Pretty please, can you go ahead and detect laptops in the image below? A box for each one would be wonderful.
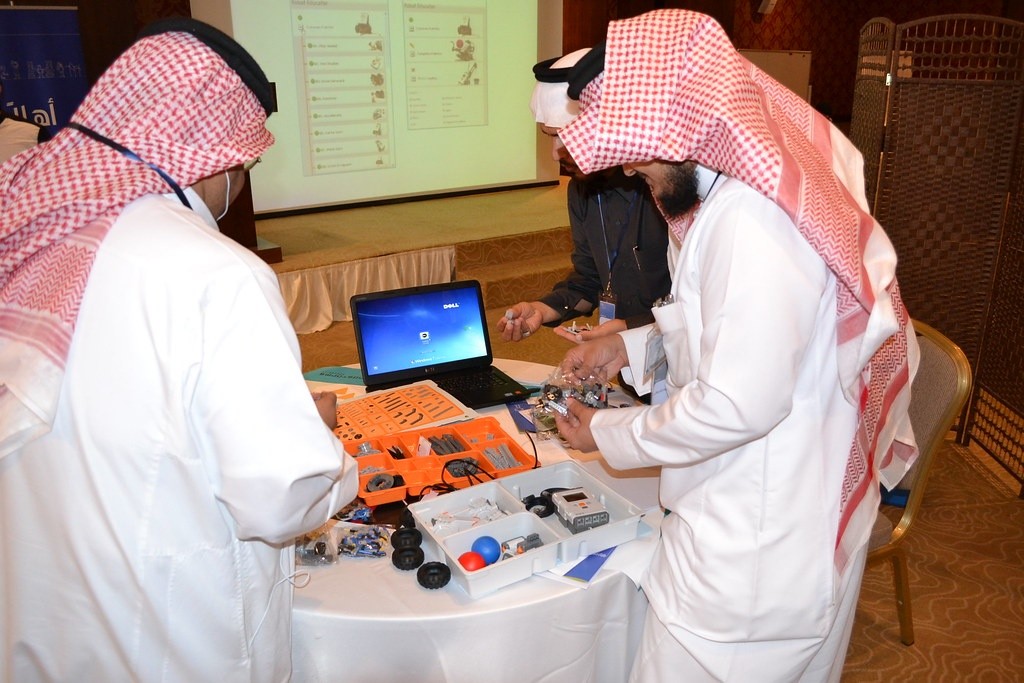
[349,280,531,410]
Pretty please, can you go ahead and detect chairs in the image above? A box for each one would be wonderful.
[862,319,972,650]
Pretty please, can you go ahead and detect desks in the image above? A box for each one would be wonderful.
[286,357,663,683]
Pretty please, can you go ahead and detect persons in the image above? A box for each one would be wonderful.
[0,15,359,683]
[498,41,673,344]
[556,9,920,683]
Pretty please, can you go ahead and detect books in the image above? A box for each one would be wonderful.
[534,546,617,590]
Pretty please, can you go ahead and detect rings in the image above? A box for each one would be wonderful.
[521,329,532,337]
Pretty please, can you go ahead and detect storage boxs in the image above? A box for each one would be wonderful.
[342,415,541,507]
[406,459,645,597]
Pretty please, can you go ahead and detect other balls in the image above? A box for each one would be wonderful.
[458,551,486,571]
[472,536,501,565]
